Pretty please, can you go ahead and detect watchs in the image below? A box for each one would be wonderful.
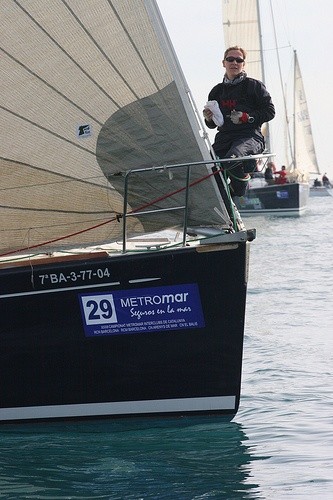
[247,113,255,123]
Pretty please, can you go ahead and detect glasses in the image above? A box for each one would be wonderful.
[225,56,245,63]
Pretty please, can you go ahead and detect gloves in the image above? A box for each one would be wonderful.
[230,110,249,125]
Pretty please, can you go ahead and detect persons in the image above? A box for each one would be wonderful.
[265,163,275,185]
[314,178,321,185]
[272,166,287,184]
[322,173,328,185]
[202,45,276,198]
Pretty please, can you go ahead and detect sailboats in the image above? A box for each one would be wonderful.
[0,0,333,428]
